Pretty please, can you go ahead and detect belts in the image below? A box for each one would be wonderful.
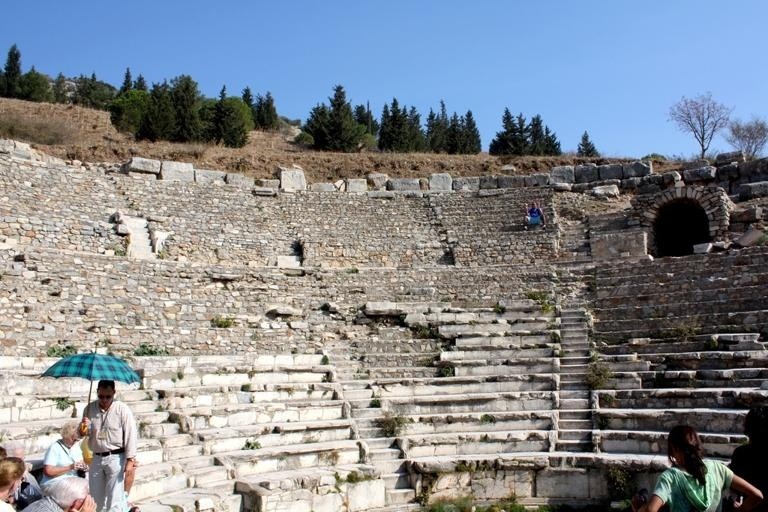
[96,447,125,456]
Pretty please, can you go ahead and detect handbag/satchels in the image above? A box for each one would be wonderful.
[17,482,42,510]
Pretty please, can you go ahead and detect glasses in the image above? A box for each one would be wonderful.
[98,394,113,399]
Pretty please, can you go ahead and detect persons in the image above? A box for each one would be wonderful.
[524,200,547,231]
[728,404,768,511]
[628,495,648,512]
[647,423,764,512]
[0,379,139,512]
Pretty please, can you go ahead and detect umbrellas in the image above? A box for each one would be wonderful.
[39,345,143,435]
